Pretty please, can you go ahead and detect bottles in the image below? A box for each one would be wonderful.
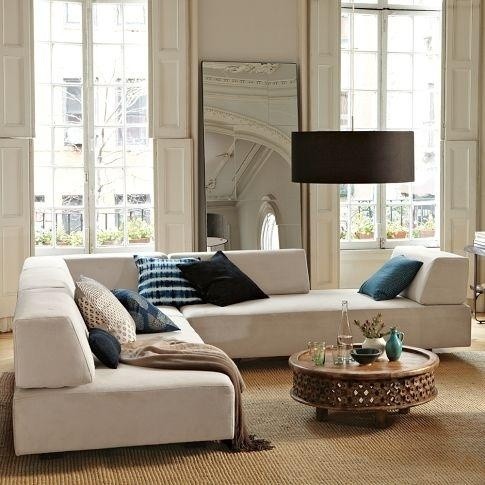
[338,301,352,362]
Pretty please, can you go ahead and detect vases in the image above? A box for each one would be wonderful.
[361,338,387,358]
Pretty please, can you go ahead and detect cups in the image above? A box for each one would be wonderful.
[308,339,323,361]
[344,349,356,364]
[314,342,326,366]
[331,346,346,365]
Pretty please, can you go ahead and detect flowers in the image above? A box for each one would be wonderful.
[352,313,398,338]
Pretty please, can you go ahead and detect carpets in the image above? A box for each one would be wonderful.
[0,351,485,485]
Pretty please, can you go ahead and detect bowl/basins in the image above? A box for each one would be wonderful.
[349,348,382,364]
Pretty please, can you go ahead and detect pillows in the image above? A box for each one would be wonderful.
[174,250,272,308]
[131,255,207,307]
[106,287,182,336]
[356,253,425,303]
[74,273,138,345]
[86,326,122,370]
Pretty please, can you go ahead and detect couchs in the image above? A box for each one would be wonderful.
[13,251,237,461]
[169,246,473,361]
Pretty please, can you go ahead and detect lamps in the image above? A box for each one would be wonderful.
[207,211,230,247]
[290,1,416,186]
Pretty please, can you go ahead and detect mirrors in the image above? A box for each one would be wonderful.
[195,57,311,291]
